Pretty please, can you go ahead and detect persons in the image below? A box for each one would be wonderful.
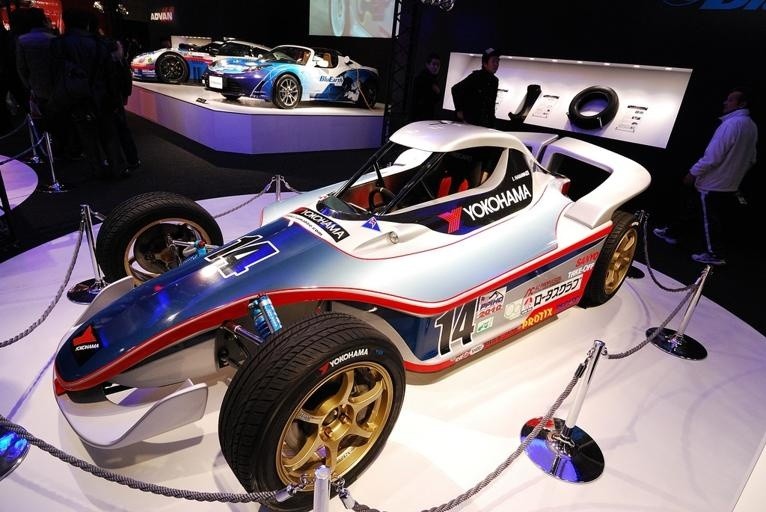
[406,54,443,125]
[652,87,759,267]
[45,7,142,179]
[451,46,503,123]
[13,6,61,162]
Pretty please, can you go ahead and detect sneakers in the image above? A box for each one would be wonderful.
[692,252,728,265]
[653,227,678,245]
[119,159,144,177]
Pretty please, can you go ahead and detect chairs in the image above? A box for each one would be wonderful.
[301,50,334,68]
[419,147,482,203]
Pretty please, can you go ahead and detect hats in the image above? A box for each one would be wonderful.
[483,48,502,64]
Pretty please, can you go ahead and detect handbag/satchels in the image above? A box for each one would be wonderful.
[104,57,132,98]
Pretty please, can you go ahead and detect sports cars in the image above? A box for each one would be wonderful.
[129,37,379,110]
[53,120,652,512]
